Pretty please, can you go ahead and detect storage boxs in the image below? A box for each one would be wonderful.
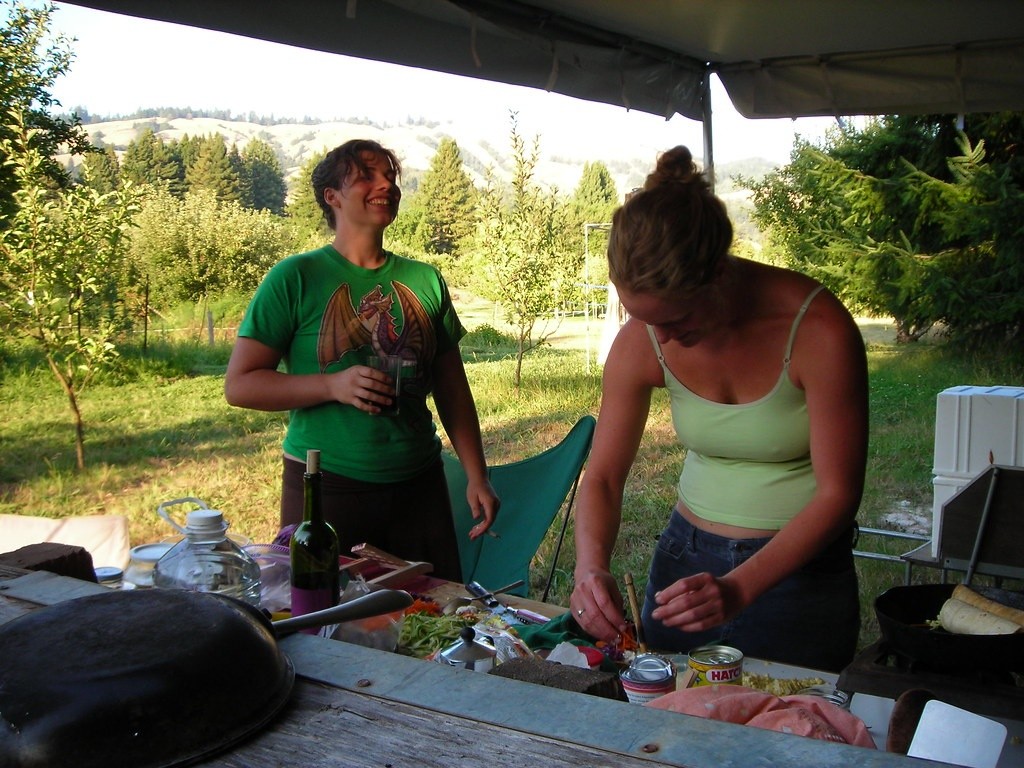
[932,385,1024,562]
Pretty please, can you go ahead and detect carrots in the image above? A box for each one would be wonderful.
[356,598,439,629]
[595,623,636,653]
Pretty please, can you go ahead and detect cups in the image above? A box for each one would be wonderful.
[370,354,404,416]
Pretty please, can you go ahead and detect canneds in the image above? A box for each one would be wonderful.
[618,652,677,705]
[686,645,744,688]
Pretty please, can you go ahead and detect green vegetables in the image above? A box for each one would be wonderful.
[393,608,475,659]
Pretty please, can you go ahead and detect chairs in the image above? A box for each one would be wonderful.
[437,414,595,602]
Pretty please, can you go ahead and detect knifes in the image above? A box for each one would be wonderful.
[464,579,522,623]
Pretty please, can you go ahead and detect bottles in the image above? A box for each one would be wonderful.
[152,497,262,611]
[290,448,342,635]
[95,566,123,589]
[122,543,175,589]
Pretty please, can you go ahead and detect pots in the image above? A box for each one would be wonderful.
[0,588,416,767]
[874,584,1024,680]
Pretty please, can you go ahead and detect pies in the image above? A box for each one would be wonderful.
[938,583,1024,634]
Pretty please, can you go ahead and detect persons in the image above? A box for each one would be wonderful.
[569,146,868,672]
[224,139,501,584]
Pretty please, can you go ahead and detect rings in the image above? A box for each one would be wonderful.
[577,609,585,617]
